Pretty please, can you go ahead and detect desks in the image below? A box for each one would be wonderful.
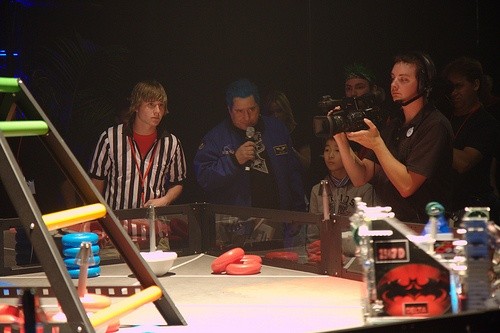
[0,201,500,333]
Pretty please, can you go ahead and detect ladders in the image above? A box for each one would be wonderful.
[0,75,189,331]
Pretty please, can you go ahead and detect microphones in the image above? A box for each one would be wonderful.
[395,93,425,107]
[245,127,255,171]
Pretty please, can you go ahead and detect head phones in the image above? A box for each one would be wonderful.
[415,52,434,93]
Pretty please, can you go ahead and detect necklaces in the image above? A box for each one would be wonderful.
[454,105,479,139]
[127,133,158,207]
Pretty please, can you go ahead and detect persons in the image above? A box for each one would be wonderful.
[261,90,311,173]
[193,79,308,250]
[5,65,76,209]
[327,50,455,224]
[90,78,186,240]
[443,57,499,226]
[309,136,379,255]
[344,64,391,160]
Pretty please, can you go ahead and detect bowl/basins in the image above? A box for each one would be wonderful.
[139,252,176,276]
